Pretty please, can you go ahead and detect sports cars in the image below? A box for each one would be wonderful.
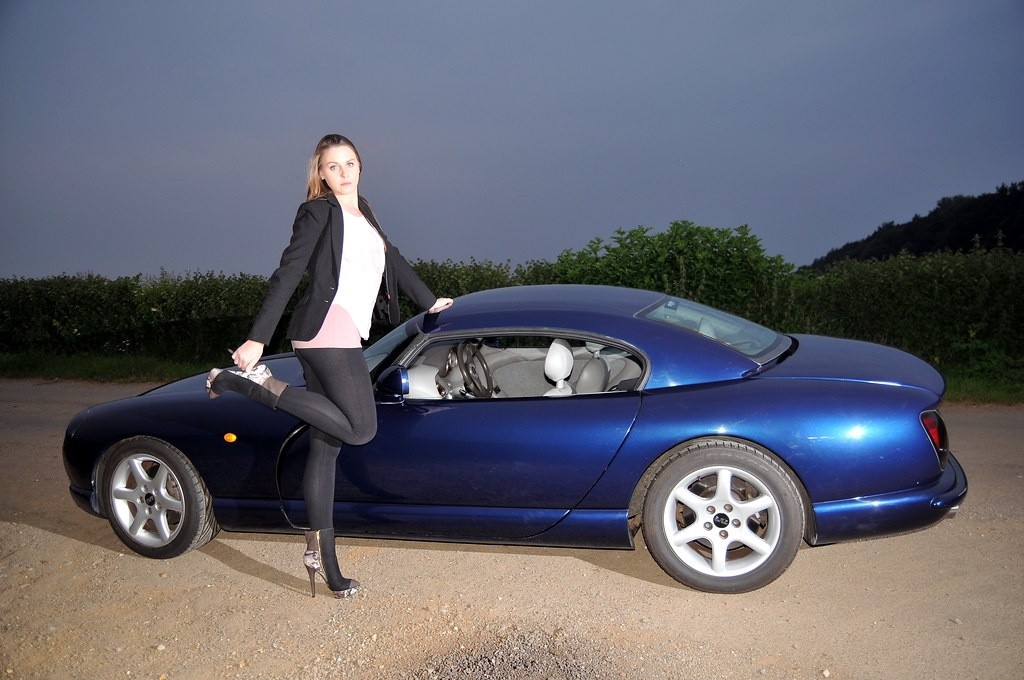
[58,284,970,594]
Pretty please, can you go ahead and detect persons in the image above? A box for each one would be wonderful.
[204,135,454,595]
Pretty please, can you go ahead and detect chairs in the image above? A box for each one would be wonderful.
[575,342,609,393]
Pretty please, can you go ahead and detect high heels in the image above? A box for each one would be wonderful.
[205,348,289,411]
[305,528,360,599]
